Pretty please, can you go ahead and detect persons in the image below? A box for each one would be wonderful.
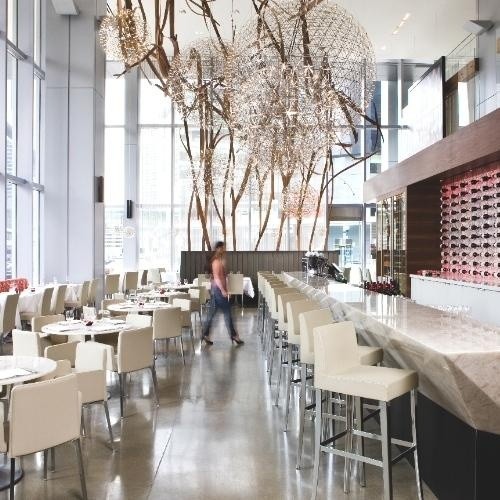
[200,240,244,345]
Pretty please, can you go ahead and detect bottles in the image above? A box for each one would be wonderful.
[438,172,500,279]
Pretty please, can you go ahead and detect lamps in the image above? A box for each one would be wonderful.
[97,174,105,203]
[127,199,132,218]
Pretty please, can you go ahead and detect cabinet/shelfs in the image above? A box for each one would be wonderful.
[376,185,442,298]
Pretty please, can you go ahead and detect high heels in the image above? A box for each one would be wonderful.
[201,335,213,345]
[231,333,245,345]
